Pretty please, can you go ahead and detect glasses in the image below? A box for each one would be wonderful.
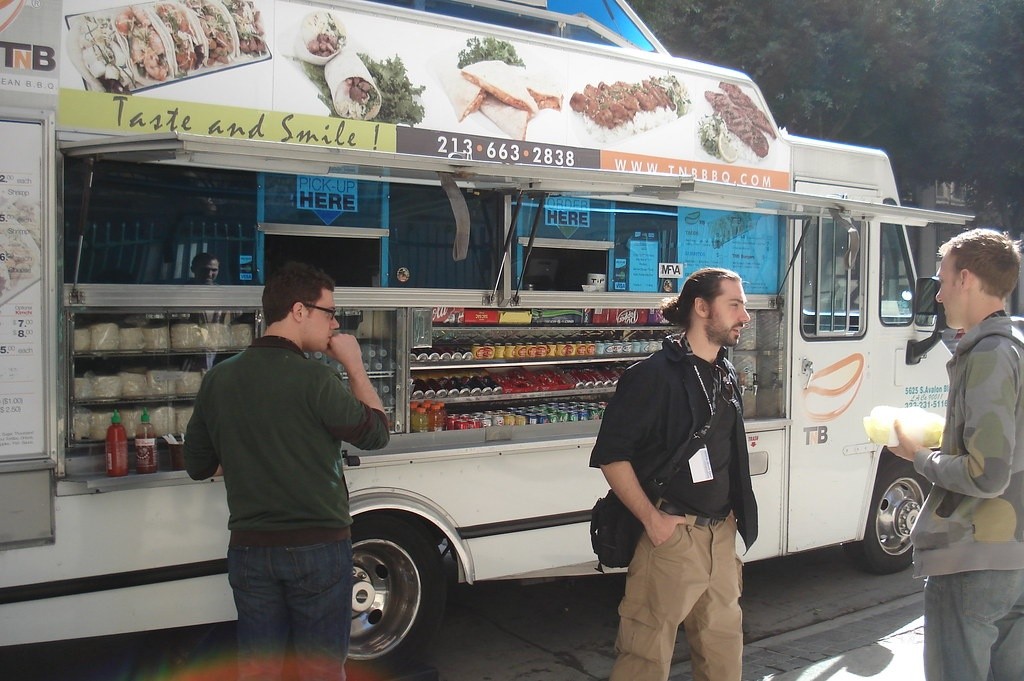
[302,303,337,320]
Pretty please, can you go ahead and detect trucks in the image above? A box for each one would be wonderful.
[1,0,1019,667]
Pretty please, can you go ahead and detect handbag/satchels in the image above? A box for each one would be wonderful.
[590,487,648,573]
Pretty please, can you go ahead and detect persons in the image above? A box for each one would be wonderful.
[590,268,760,681]
[175,255,232,372]
[182,264,391,681]
[885,227,1024,680]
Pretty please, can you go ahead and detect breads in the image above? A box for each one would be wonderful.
[443,55,562,142]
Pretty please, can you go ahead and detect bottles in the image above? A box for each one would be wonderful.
[105,408,128,478]
[303,342,397,410]
[134,407,158,474]
[409,400,445,434]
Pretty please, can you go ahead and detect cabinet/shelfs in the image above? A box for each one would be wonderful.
[62,309,404,458]
[404,304,684,439]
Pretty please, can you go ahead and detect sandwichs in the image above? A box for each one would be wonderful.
[65,0,266,93]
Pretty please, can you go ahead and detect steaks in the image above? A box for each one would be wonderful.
[706,79,776,158]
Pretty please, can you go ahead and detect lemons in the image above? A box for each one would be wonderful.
[716,132,738,163]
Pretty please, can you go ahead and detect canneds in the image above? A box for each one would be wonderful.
[411,333,677,437]
